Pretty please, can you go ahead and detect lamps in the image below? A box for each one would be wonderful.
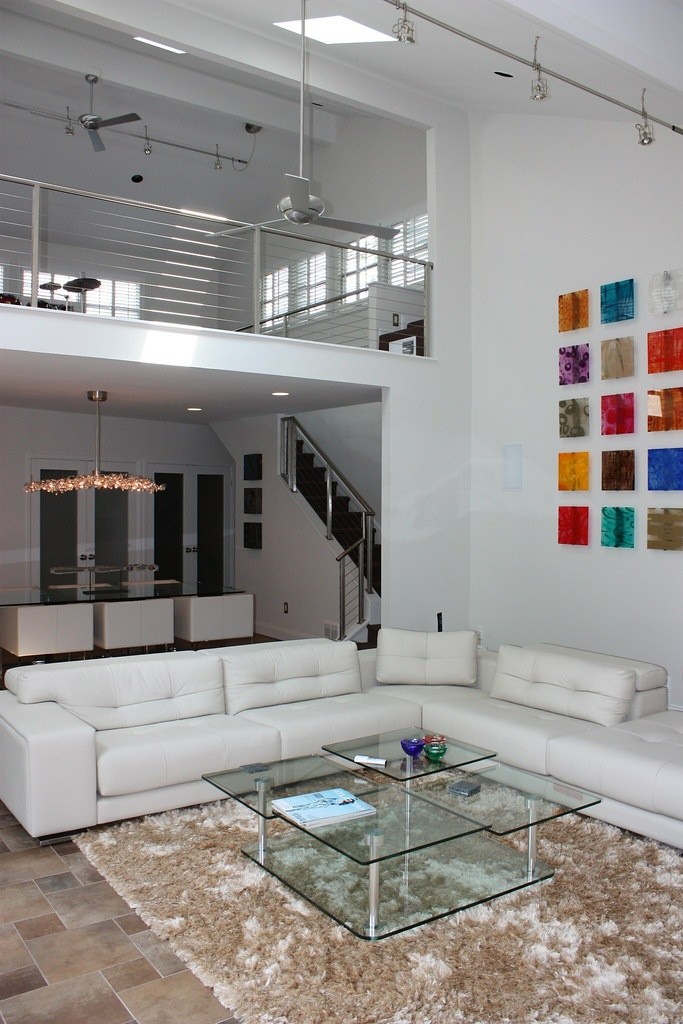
[64,107,74,136]
[529,35,549,101]
[392,0,415,44]
[144,126,152,154]
[215,144,222,170]
[635,89,656,145]
[23,391,167,494]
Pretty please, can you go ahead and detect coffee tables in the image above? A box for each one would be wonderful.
[201,725,601,942]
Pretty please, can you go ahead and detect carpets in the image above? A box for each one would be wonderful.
[71,756,683,1024]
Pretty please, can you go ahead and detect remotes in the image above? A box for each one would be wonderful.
[354,756,387,765]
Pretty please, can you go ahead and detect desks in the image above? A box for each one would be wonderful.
[0,582,245,606]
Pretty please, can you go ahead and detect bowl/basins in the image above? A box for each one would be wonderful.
[400,735,448,761]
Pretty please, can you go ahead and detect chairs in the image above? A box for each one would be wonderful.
[93,599,174,659]
[172,593,254,650]
[0,603,94,665]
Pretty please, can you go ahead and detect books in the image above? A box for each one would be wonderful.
[270,788,377,829]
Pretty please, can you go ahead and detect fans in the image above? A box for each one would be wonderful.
[31,75,141,152]
[205,1,399,240]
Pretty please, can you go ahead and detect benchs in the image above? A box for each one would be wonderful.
[0,627,683,852]
